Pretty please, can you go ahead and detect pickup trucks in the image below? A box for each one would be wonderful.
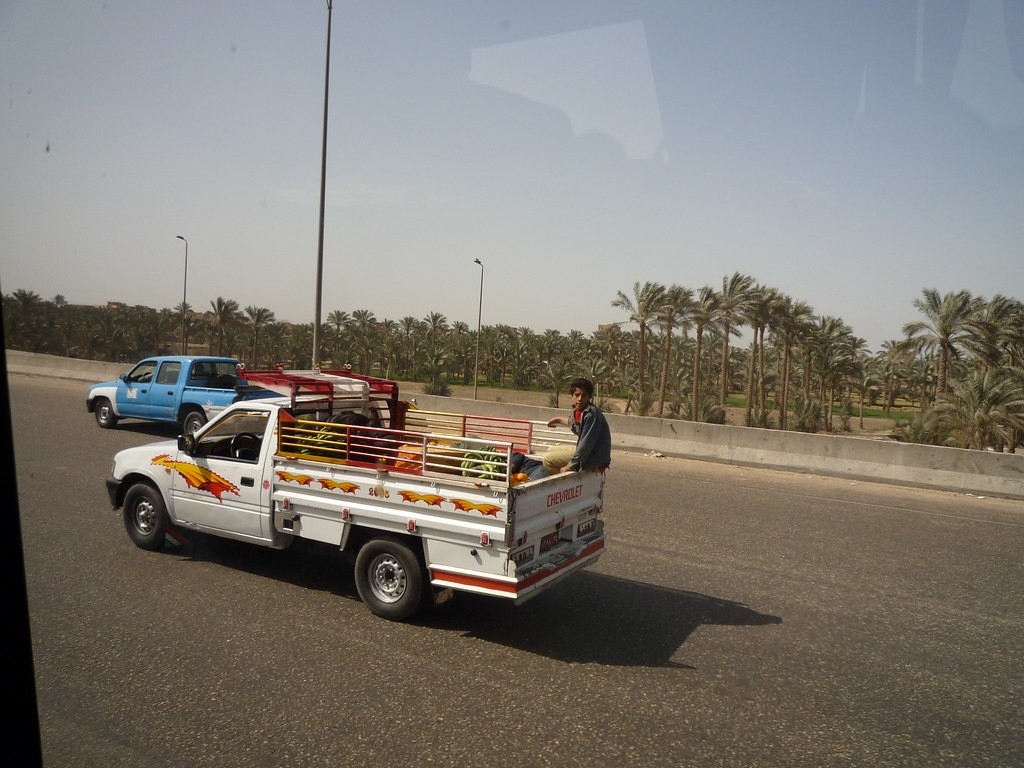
[85,356,286,441]
[105,363,608,622]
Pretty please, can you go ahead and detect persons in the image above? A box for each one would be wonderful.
[543,378,611,475]
[501,452,549,481]
[341,414,395,464]
[194,364,204,375]
[138,363,167,383]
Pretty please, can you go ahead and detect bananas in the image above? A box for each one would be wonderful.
[297,413,347,455]
[461,445,507,481]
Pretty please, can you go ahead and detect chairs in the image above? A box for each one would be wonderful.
[163,370,179,383]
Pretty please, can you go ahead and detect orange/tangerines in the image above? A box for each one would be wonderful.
[509,472,528,485]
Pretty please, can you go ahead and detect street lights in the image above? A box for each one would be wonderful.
[473,258,484,400]
[176,235,188,356]
